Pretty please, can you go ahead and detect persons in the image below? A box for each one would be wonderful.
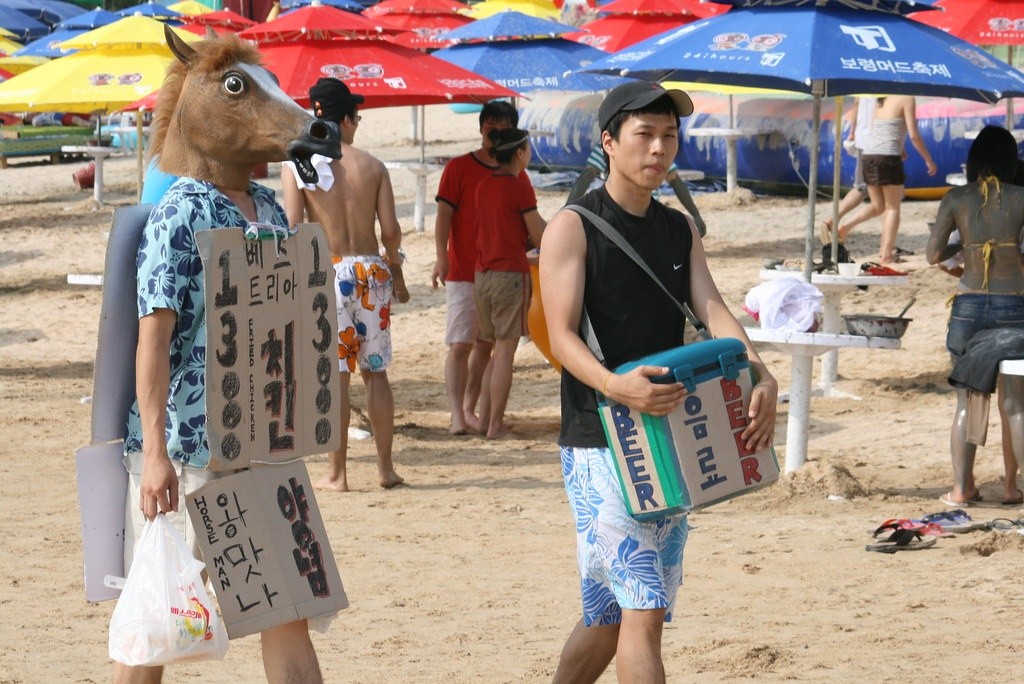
[820,96,899,259]
[113,176,326,684]
[281,78,405,491]
[828,96,936,264]
[23,112,94,126]
[474,128,546,440]
[566,142,706,239]
[539,84,778,683]
[431,100,548,434]
[926,125,1024,507]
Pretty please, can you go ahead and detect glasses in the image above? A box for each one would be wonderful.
[343,113,362,122]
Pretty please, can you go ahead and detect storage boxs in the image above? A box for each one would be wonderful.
[596,340,779,525]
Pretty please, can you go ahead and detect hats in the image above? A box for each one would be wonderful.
[309,77,366,118]
[598,81,694,132]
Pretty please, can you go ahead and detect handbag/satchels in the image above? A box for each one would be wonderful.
[101,513,232,670]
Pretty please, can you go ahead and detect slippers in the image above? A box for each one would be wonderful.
[820,222,831,244]
[865,507,1024,554]
[894,257,907,262]
[838,233,844,246]
[893,247,914,255]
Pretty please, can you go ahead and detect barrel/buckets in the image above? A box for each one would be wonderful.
[73,160,95,190]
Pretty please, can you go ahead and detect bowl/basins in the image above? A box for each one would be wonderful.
[763,257,785,269]
[842,314,913,338]
[838,263,861,276]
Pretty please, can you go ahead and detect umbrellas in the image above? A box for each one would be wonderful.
[0,0,1024,472]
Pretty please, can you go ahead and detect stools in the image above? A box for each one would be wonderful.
[687,124,775,202]
[674,169,705,185]
[61,144,117,210]
[757,264,911,387]
[741,328,901,474]
[945,172,968,185]
[384,162,444,233]
